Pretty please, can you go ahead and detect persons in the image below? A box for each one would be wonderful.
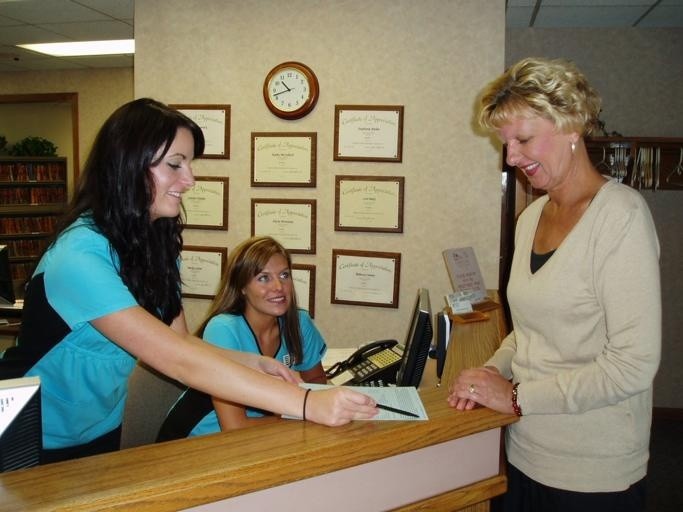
[101,236,327,444]
[445,58,662,511]
[0,98,379,464]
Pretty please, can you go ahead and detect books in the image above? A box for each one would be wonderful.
[608,142,661,192]
[0,162,67,280]
[442,247,488,304]
[0,376,42,472]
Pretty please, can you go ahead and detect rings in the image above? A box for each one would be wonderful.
[469,384,475,392]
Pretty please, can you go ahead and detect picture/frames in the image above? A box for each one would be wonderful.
[178,177,229,230]
[291,264,316,319]
[180,246,227,299]
[251,198,316,254]
[251,132,317,187]
[168,104,230,159]
[335,175,404,233]
[331,249,400,308]
[334,105,403,162]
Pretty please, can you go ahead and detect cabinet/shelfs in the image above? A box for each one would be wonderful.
[0,156,68,280]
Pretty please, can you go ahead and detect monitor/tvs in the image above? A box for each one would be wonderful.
[396,288,433,388]
[0,245,16,304]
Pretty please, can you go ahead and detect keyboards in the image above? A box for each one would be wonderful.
[352,380,383,387]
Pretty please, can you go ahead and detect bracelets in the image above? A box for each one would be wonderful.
[302,389,310,422]
[512,382,523,416]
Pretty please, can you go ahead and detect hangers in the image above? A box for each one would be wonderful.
[595,142,683,192]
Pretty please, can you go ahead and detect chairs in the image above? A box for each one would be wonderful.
[120,360,188,450]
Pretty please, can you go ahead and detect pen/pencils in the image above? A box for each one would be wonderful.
[376,404,420,418]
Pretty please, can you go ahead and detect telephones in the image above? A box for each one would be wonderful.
[340,340,405,384]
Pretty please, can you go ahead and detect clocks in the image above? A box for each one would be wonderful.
[263,62,319,120]
